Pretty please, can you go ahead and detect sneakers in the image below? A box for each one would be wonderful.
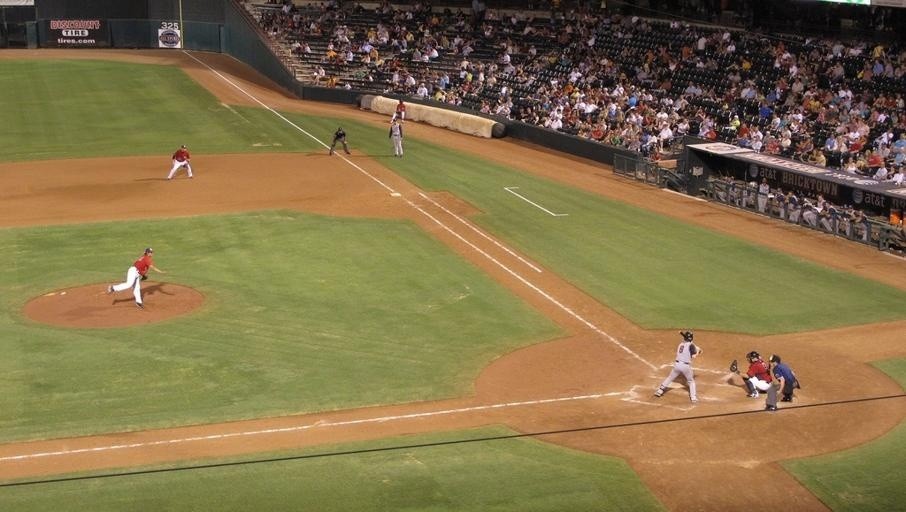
[747,393,759,397]
[655,388,664,397]
[765,406,776,411]
[781,397,790,401]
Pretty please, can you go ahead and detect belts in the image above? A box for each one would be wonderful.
[676,360,688,364]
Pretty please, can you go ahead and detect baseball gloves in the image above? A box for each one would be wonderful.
[141,273,149,279]
[730,360,738,373]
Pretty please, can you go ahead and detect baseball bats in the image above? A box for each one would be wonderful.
[679,330,702,353]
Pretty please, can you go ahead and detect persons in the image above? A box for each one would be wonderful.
[653,332,702,404]
[612,0,905,244]
[167,142,194,180]
[257,0,633,147]
[387,118,404,159]
[765,355,796,412]
[735,350,772,398]
[328,127,351,156]
[105,247,168,308]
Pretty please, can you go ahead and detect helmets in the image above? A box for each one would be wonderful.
[746,351,759,359]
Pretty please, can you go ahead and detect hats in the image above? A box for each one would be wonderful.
[766,355,780,364]
[685,331,693,341]
